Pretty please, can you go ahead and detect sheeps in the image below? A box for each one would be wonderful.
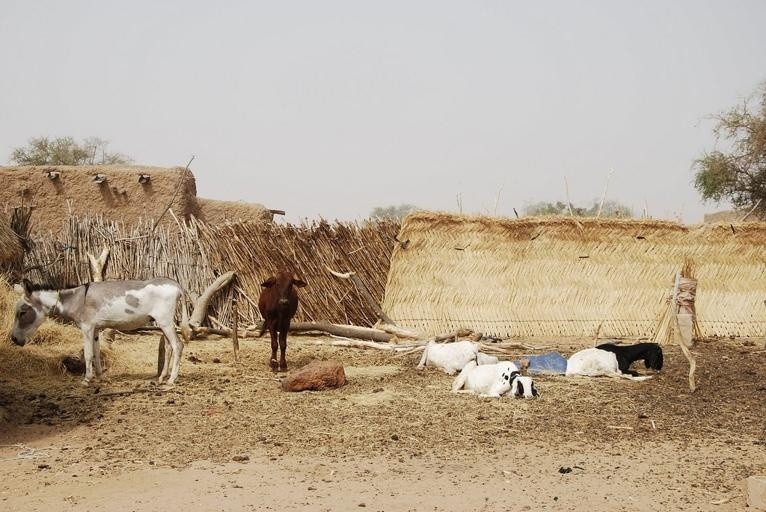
[564,341,664,383]
[415,341,500,377]
[449,359,539,400]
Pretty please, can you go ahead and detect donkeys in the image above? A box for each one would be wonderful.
[9,277,193,388]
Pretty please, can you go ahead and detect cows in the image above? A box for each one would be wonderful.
[257,271,309,372]
